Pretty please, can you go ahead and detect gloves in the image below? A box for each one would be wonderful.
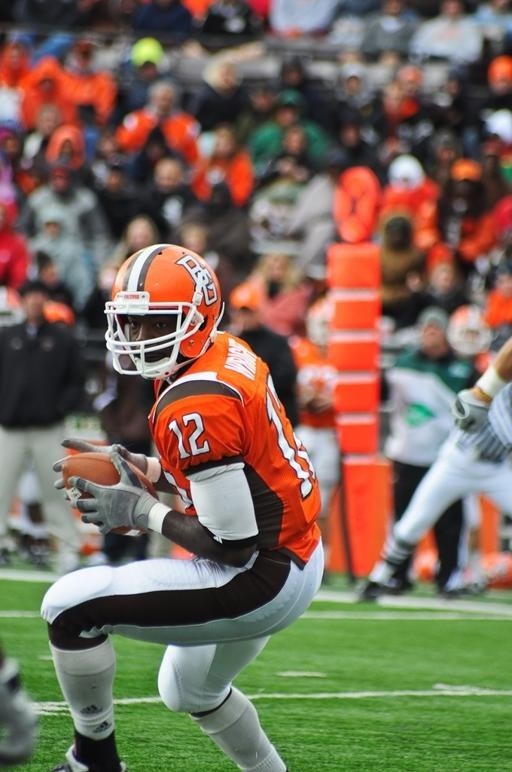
[52,438,161,500]
[68,453,172,537]
[452,389,490,433]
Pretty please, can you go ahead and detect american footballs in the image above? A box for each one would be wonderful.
[61,453,158,536]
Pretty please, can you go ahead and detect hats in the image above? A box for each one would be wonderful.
[1,0,510,185]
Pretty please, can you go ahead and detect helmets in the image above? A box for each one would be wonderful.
[102,243,226,382]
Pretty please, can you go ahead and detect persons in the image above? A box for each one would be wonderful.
[0,0,512,604]
[40,243,327,772]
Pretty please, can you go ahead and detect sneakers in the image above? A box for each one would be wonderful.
[358,558,406,600]
